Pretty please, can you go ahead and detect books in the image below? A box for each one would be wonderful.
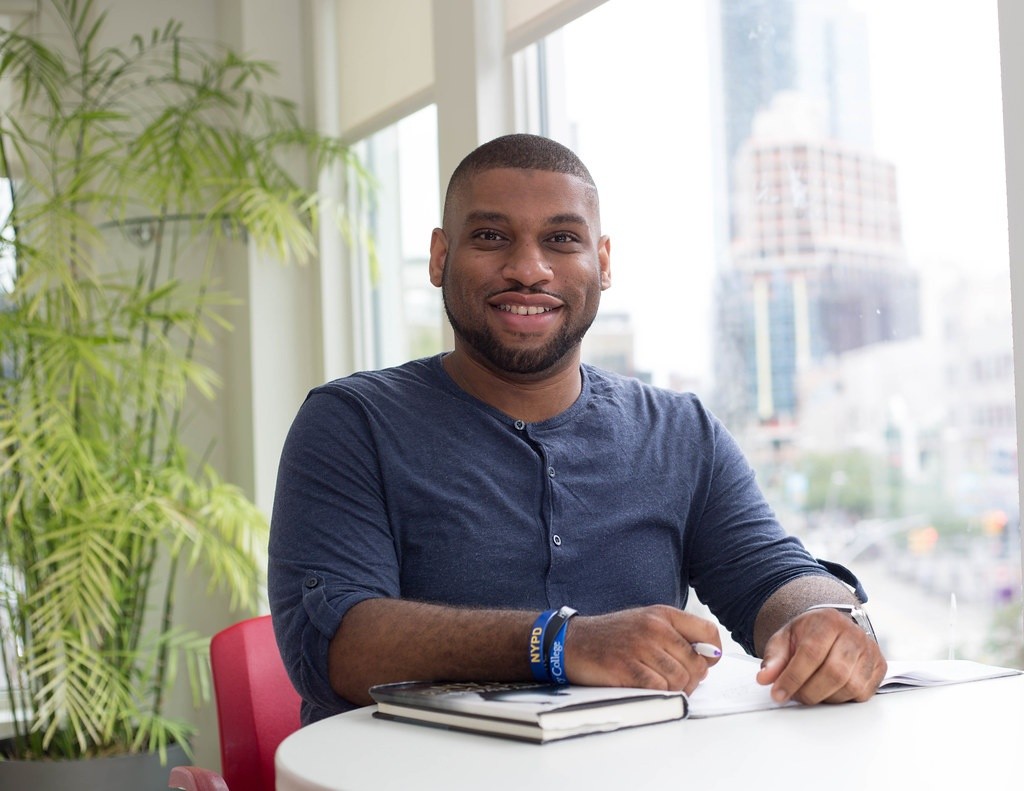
[367,680,691,746]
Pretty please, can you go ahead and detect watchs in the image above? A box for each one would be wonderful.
[804,603,879,650]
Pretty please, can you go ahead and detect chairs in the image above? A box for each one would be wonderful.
[210,615,306,791]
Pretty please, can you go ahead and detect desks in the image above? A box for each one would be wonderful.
[273,634,1024,791]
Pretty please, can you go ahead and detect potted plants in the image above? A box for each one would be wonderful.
[0,0,365,790]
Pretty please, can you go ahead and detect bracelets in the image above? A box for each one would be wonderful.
[527,605,580,690]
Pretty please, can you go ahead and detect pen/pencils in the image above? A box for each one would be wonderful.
[691,642,721,658]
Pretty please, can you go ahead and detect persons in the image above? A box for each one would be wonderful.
[265,133,889,706]
